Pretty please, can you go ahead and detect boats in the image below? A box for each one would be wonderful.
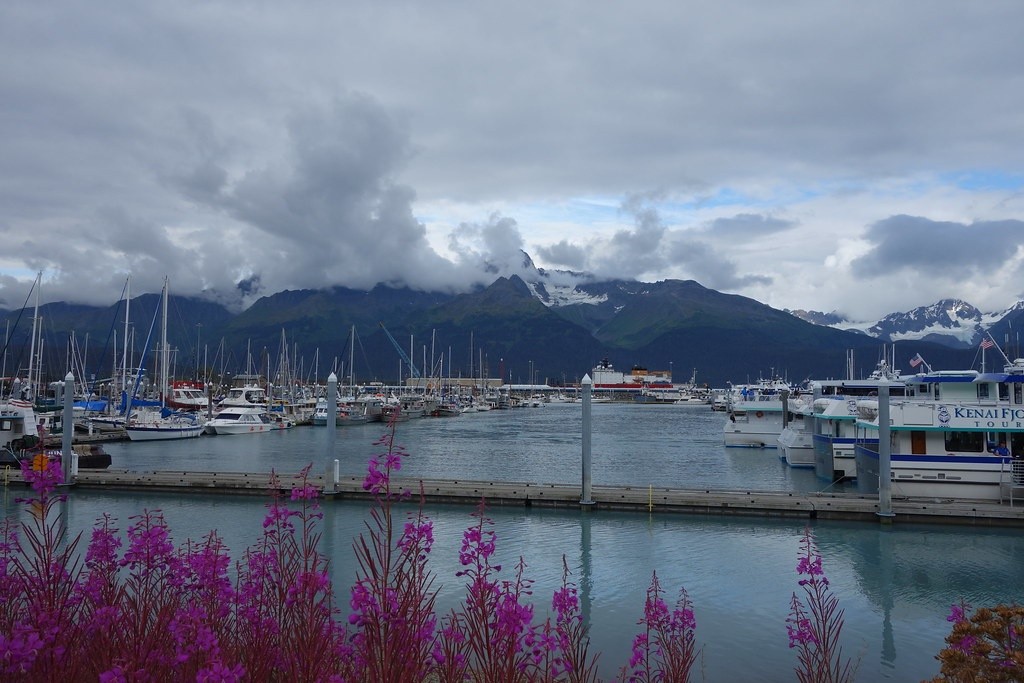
[549,393,572,403]
[590,327,1024,497]
[574,396,613,405]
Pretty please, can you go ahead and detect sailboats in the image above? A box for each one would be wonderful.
[0,260,544,468]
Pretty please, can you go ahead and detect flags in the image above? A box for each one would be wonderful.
[980,336,994,350]
[909,354,922,367]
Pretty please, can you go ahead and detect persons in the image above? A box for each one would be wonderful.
[742,387,775,401]
[993,439,1011,456]
